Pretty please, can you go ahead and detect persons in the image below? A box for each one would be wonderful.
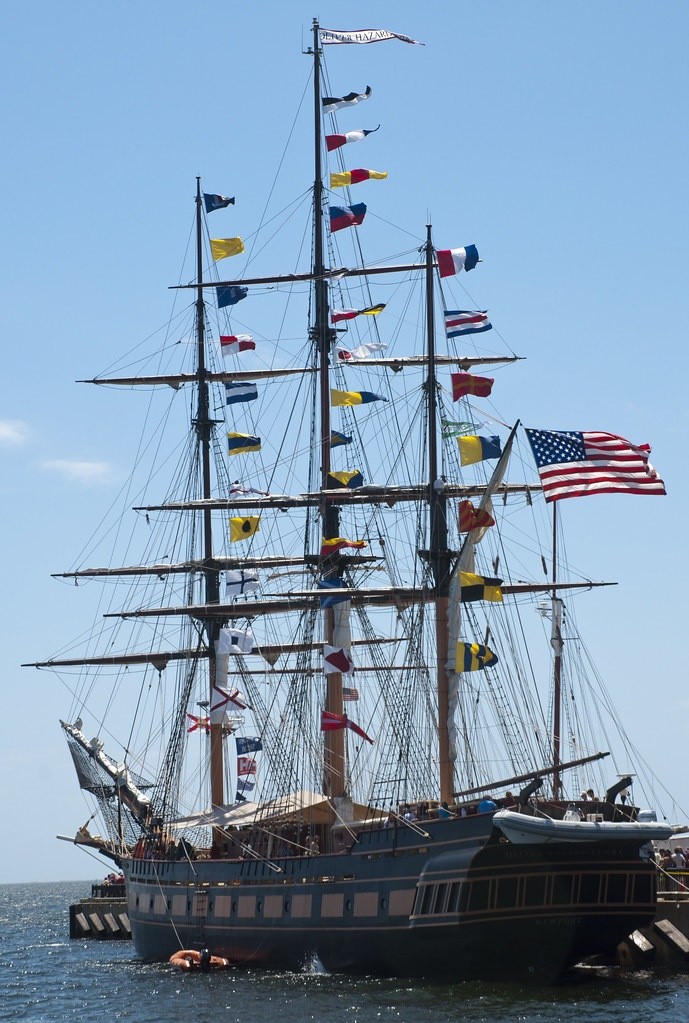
[439,802,449,818]
[242,839,251,858]
[145,837,198,861]
[477,791,515,813]
[276,841,294,857]
[384,807,415,828]
[658,846,689,891]
[103,873,125,897]
[581,789,599,801]
[305,835,319,855]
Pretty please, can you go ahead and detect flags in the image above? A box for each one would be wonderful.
[204,193,261,655]
[524,426,668,503]
[235,738,262,801]
[435,244,504,672]
[326,470,363,490]
[186,714,211,732]
[210,685,247,712]
[343,688,359,701]
[323,644,355,676]
[329,203,367,233]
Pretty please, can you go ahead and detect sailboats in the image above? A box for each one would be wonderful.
[13,18,689,1001]
